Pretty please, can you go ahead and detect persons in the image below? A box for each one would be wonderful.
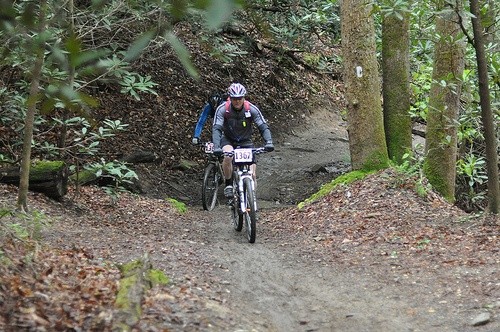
[192,89,236,185]
[212,83,274,215]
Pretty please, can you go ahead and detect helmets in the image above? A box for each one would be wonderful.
[226,83,247,97]
[208,90,223,109]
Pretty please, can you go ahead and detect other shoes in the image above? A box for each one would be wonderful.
[224,185,233,198]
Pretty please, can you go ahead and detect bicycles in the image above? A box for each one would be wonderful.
[210,142,274,244]
[193,141,230,211]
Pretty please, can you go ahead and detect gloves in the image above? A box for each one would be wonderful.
[213,144,224,157]
[264,139,274,153]
[192,138,199,144]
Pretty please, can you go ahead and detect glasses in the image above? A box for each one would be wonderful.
[232,97,242,100]
